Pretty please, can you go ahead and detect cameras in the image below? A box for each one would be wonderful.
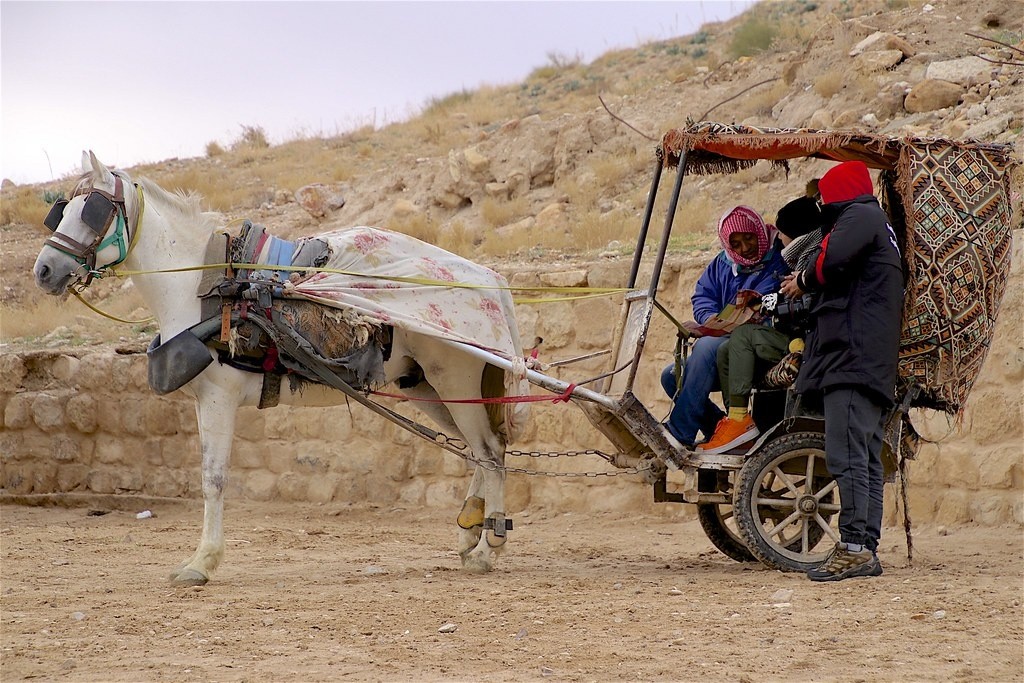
[778,291,820,328]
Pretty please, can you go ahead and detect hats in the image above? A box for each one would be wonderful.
[773,194,820,237]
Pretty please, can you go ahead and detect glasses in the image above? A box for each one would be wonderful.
[816,198,824,211]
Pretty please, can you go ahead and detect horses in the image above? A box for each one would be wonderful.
[33,148,536,588]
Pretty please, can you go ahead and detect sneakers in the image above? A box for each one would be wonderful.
[806,543,878,581]
[694,412,759,454]
[725,442,755,455]
[848,550,884,577]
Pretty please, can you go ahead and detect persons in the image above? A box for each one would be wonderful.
[658,202,792,455]
[694,194,829,458]
[771,159,901,583]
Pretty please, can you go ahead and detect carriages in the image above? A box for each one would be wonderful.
[33,117,1020,589]
